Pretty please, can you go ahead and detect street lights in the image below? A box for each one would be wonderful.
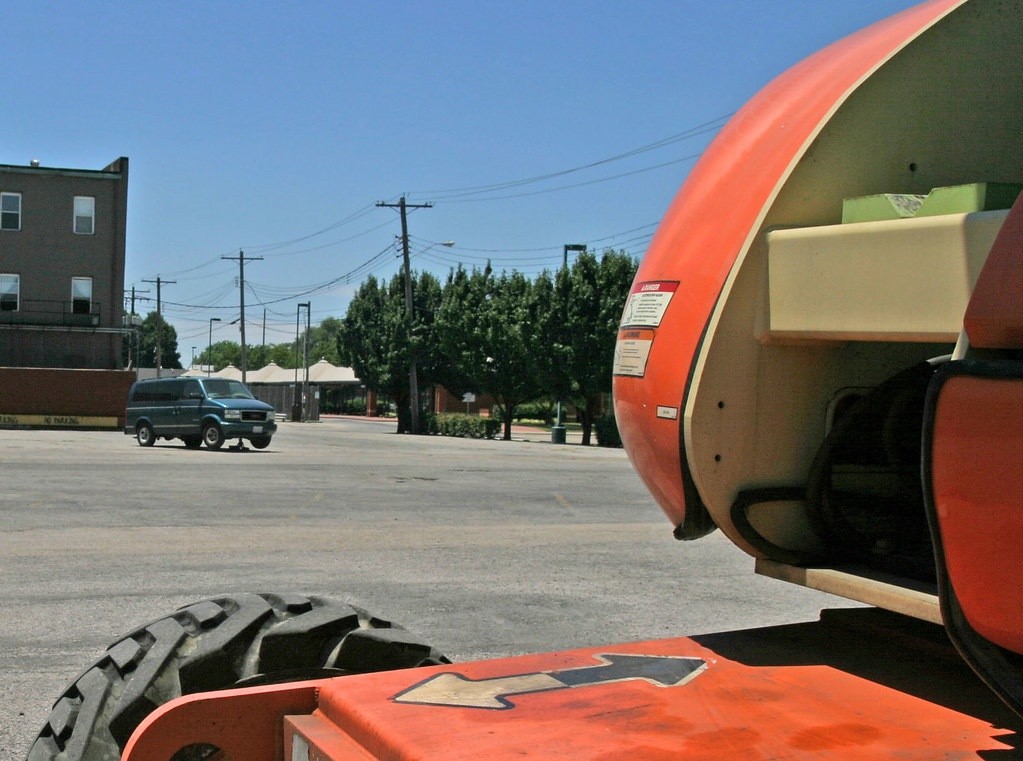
[192,346,196,369]
[207,318,222,378]
[405,240,457,433]
[291,303,308,421]
[557,245,587,425]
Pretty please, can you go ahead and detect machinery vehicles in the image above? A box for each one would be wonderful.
[20,1,1021,761]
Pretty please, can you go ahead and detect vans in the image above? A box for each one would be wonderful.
[124,376,279,449]
